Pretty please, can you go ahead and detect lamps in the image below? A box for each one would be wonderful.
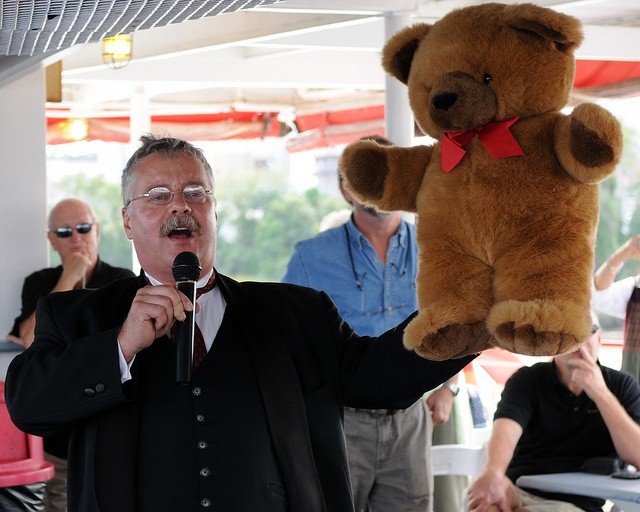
[101,33,134,70]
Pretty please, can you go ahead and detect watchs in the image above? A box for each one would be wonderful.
[442,382,460,396]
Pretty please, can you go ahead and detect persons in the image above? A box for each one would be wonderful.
[462,311,640,512]
[594,235,640,382]
[6,198,136,511]
[281,135,461,512]
[6,136,483,511]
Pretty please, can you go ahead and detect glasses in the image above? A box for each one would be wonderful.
[592,326,600,334]
[125,186,214,206]
[359,271,415,318]
[51,223,93,237]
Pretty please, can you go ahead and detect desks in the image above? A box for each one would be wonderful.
[516,472,640,512]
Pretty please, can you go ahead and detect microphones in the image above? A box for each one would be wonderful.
[171,251,202,386]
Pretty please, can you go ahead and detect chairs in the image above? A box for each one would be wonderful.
[431,442,488,512]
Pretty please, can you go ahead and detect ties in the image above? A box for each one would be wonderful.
[142,273,217,366]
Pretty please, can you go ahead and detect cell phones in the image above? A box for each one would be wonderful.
[611,471,640,479]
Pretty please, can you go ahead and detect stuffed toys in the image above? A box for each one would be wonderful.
[339,4,623,360]
